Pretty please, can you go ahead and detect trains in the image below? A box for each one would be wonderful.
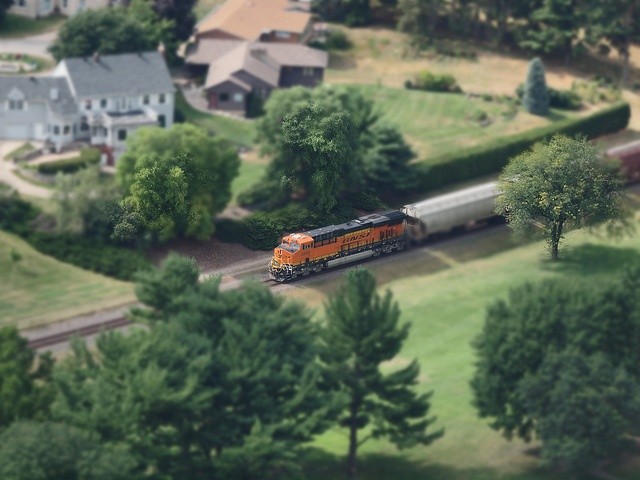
[266,140,639,284]
